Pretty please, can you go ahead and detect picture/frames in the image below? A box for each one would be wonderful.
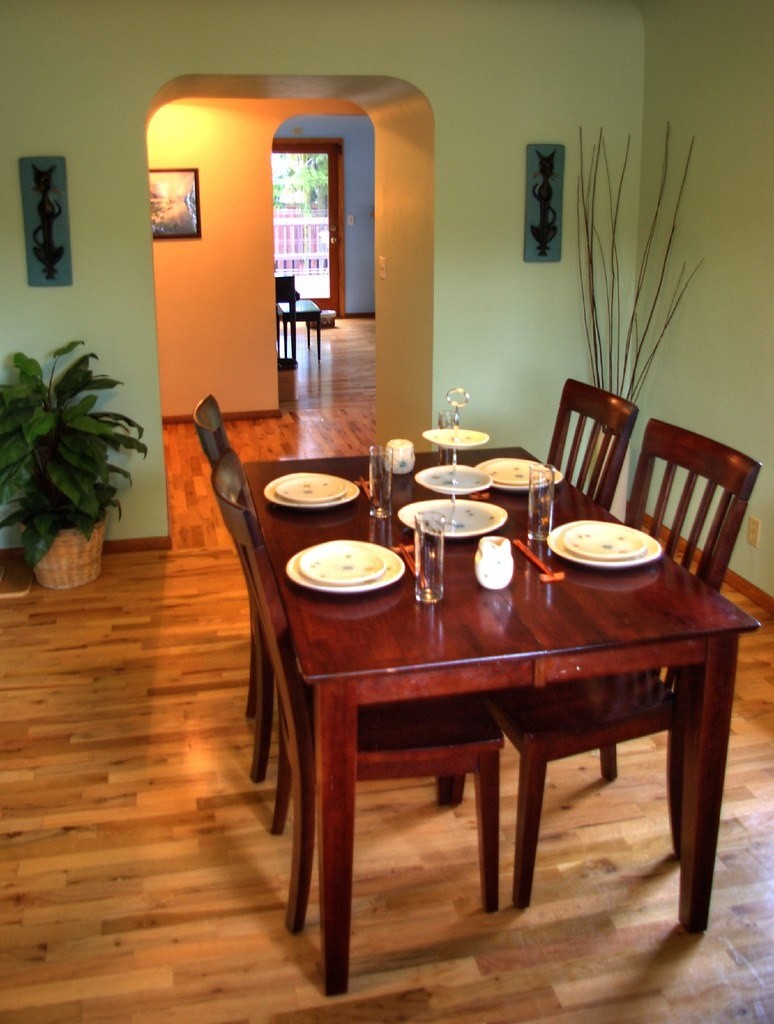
[150,167,202,239]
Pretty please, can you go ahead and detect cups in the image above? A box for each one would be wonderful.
[413,511,446,603]
[474,536,514,590]
[438,411,454,465]
[369,445,392,518]
[386,440,415,474]
[527,463,555,541]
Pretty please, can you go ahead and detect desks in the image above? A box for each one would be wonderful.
[274,274,300,360]
[239,444,753,995]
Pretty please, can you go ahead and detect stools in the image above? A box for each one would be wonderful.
[277,301,324,360]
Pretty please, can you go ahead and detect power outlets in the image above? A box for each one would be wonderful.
[746,517,761,545]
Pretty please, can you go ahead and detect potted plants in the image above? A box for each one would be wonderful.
[0,337,147,591]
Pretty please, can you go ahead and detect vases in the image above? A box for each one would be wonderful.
[598,426,630,525]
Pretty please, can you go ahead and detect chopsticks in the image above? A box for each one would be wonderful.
[513,539,553,577]
[358,476,370,499]
[398,543,428,588]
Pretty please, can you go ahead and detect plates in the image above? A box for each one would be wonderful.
[422,429,489,449]
[299,545,387,584]
[564,524,646,560]
[276,478,347,502]
[414,465,493,495]
[264,473,360,508]
[547,520,662,568]
[474,458,563,490]
[398,499,508,539]
[286,540,406,593]
[486,461,529,484]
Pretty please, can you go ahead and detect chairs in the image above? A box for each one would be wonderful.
[208,447,504,932]
[194,395,310,779]
[482,417,760,911]
[547,377,635,511]
